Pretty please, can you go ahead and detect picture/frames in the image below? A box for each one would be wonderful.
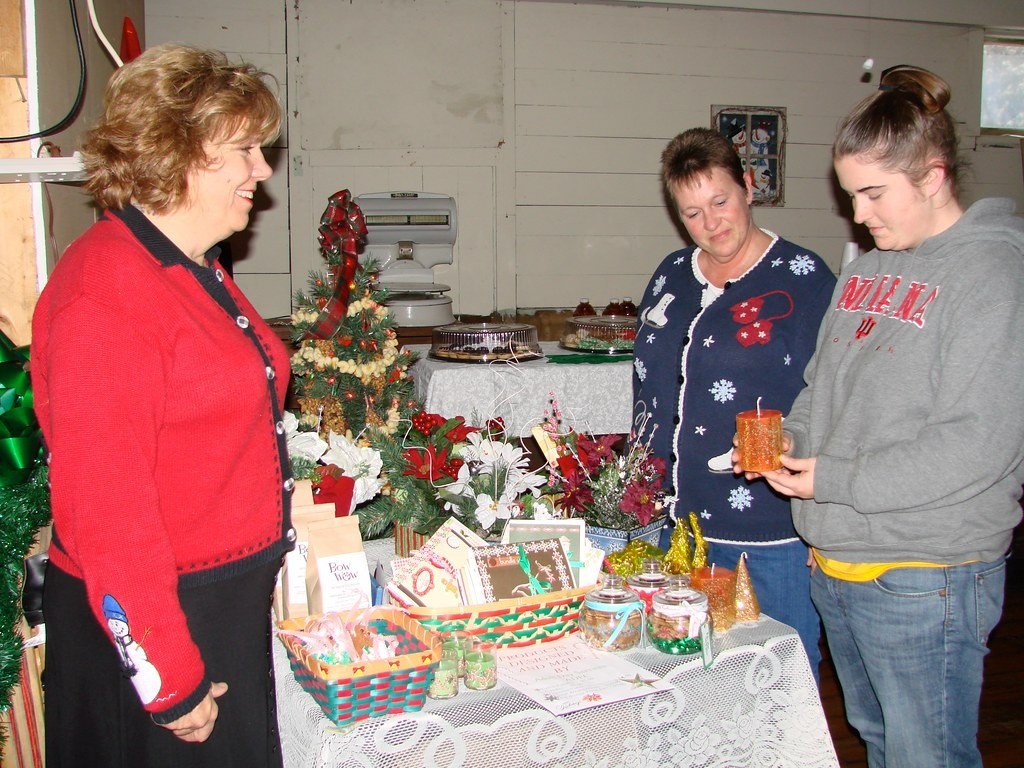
[710,104,787,208]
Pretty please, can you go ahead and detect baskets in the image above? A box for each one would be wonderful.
[279,604,442,725]
[372,568,606,653]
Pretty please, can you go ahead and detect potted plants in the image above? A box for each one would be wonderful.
[394,519,431,558]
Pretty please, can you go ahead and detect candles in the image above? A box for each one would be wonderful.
[736,397,784,472]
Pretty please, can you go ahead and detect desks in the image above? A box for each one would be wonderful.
[401,341,634,438]
[272,610,842,768]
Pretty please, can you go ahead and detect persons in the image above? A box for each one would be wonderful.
[30,42,292,768]
[731,63,1024,768]
[630,126,838,698]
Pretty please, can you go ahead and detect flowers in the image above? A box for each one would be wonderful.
[283,410,328,463]
[538,391,668,528]
[321,428,389,516]
[434,431,548,529]
[390,412,483,520]
[286,188,428,426]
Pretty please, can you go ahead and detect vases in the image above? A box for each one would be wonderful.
[586,514,668,555]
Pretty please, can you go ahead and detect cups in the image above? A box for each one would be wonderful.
[463,639,497,690]
[440,629,473,678]
[427,648,458,700]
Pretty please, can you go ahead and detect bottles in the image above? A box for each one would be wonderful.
[602,298,620,317]
[573,297,597,317]
[620,297,637,317]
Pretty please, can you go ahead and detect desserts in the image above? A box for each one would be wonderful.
[435,336,531,360]
[563,328,638,350]
